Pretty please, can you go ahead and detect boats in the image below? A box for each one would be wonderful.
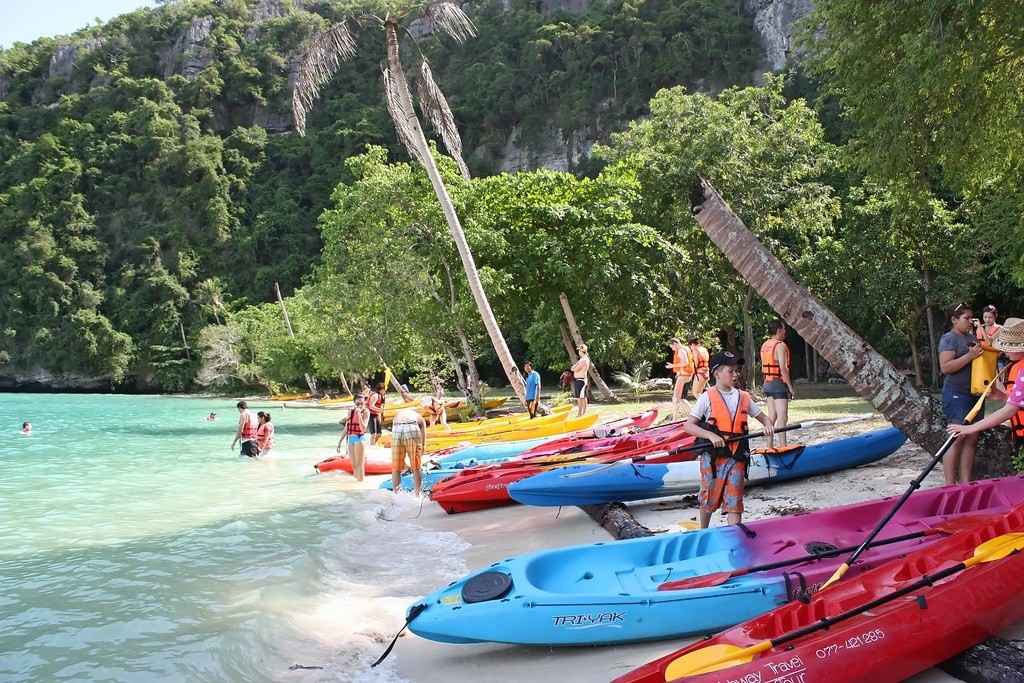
[234,390,696,518]
[506,421,909,507]
[315,395,355,404]
[405,474,1024,649]
[610,499,1024,683]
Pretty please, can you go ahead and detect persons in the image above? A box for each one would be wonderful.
[938,301,1002,486]
[571,344,590,418]
[560,369,572,393]
[19,422,32,434]
[366,384,385,444]
[434,376,445,399]
[946,318,1024,479]
[392,408,425,497]
[420,397,451,432]
[361,384,372,401]
[761,319,795,450]
[231,401,259,460]
[665,337,693,422]
[203,413,219,421]
[523,361,541,420]
[971,305,1006,399]
[684,351,774,529]
[337,393,371,482]
[257,411,274,456]
[688,338,710,400]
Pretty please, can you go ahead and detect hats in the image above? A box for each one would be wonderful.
[576,344,588,353]
[710,351,745,374]
[991,317,1024,352]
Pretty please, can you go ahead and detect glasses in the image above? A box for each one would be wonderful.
[954,302,967,312]
[355,402,363,406]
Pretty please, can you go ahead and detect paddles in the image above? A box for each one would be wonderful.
[558,419,858,479]
[575,402,670,434]
[664,531,1024,683]
[605,420,687,438]
[656,513,992,591]
[481,425,540,436]
[816,360,1014,591]
[500,440,639,469]
[377,368,391,447]
